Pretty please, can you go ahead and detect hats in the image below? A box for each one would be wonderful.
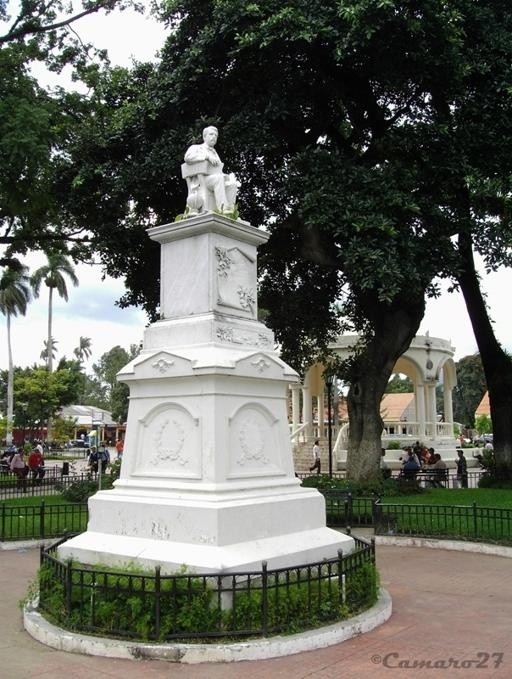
[473,432,495,445]
[64,439,91,447]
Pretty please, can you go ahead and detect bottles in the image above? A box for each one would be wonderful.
[90,447,96,450]
[457,449,464,453]
[427,448,434,451]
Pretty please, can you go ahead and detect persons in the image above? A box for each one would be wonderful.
[458,434,465,447]
[474,440,494,458]
[116,439,124,458]
[380,448,392,479]
[310,441,321,473]
[0,440,45,493]
[85,444,110,474]
[402,441,446,488]
[455,450,468,488]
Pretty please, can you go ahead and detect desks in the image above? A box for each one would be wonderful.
[323,368,336,478]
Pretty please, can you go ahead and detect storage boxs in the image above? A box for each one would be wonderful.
[398,468,450,488]
